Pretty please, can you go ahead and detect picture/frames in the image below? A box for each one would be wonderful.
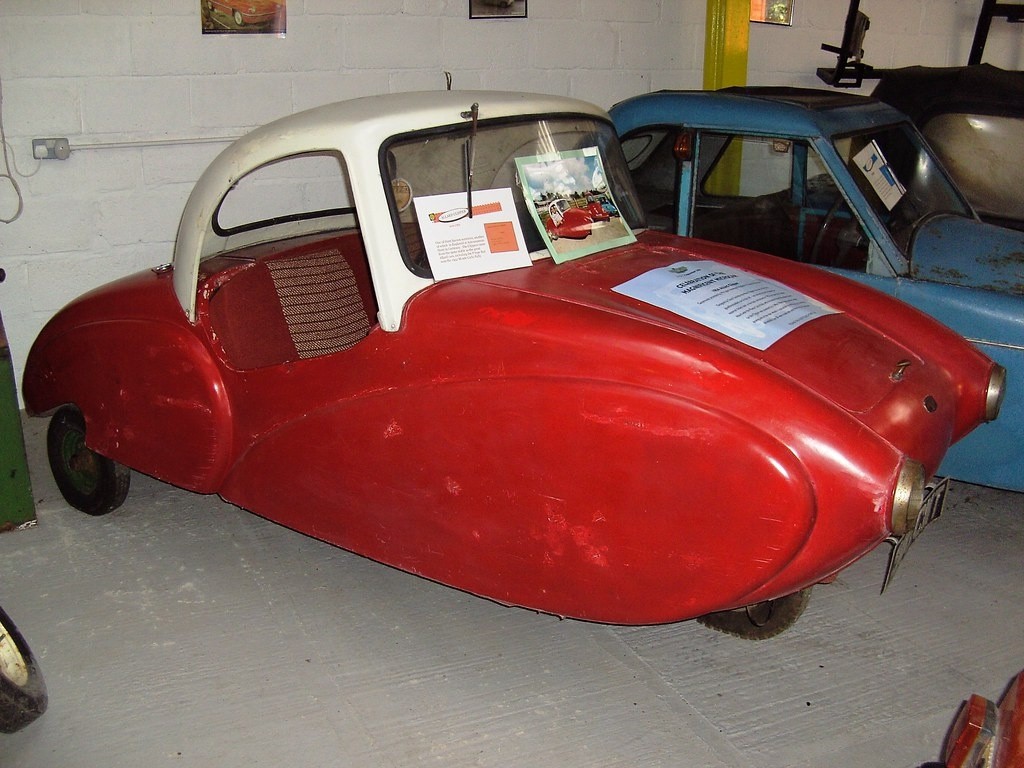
[467,0,527,19]
[750,1,795,27]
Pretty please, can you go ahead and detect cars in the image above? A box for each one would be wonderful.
[20,86,1005,644]
[569,83,1024,498]
